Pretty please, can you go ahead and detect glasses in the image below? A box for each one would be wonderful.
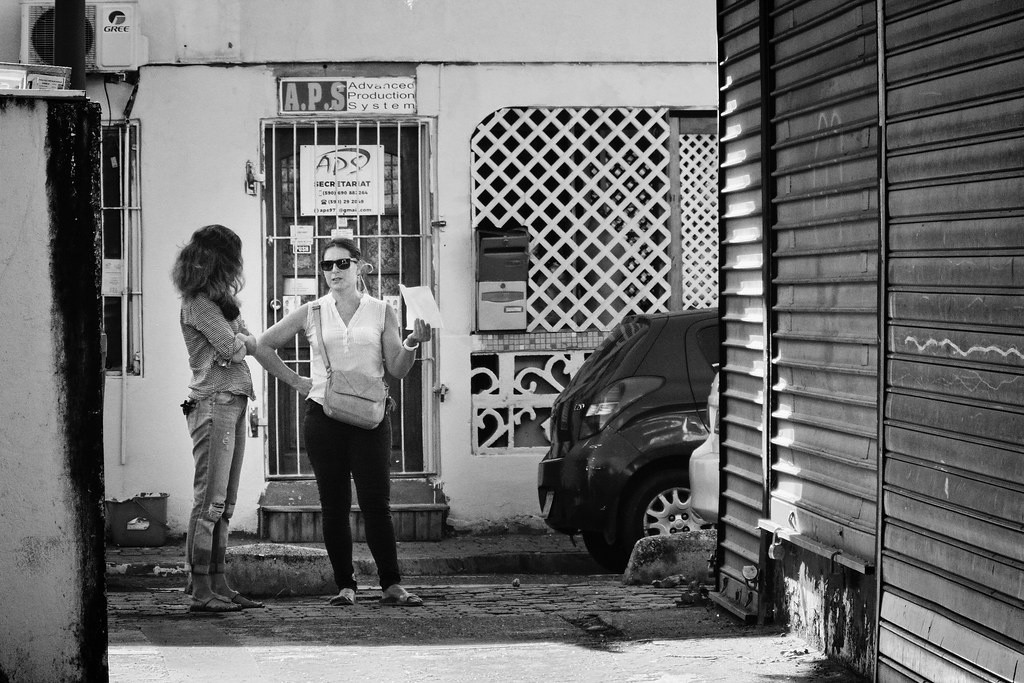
[319,258,357,271]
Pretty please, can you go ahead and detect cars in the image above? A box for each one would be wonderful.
[687,363,721,524]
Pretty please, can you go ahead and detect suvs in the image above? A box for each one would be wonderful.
[536,307,721,574]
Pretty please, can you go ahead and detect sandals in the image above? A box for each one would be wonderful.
[229,590,265,609]
[189,595,241,612]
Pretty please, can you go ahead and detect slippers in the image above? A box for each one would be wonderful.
[331,596,357,606]
[379,592,424,607]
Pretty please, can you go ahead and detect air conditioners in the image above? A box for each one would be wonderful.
[20,0,149,73]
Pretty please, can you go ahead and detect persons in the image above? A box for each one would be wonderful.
[172,225,266,611]
[253,238,431,607]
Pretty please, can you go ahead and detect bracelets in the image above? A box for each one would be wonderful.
[403,339,420,352]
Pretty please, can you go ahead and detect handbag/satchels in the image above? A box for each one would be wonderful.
[323,371,387,429]
